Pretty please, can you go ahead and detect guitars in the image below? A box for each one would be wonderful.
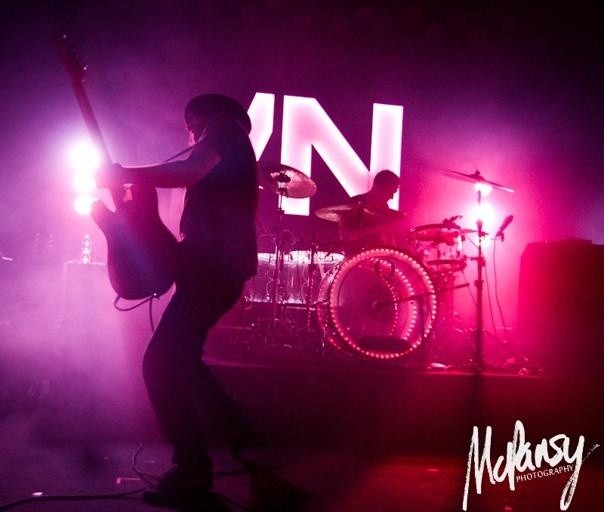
[54,22,178,301]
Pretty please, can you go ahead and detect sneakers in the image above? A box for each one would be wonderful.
[142,483,209,507]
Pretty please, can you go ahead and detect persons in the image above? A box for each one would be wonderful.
[93,90,258,505]
[337,171,403,245]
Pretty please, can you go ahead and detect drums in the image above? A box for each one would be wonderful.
[314,247,440,369]
[316,247,345,307]
[405,223,464,266]
[242,248,279,308]
[282,249,315,311]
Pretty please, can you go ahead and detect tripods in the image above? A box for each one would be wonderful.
[231,187,326,354]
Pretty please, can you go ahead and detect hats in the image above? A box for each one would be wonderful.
[184,93,252,138]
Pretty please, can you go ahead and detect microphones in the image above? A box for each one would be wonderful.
[496,215,513,237]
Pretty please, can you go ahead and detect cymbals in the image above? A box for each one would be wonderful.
[443,169,517,193]
[259,162,317,199]
[314,202,386,224]
[452,228,481,233]
